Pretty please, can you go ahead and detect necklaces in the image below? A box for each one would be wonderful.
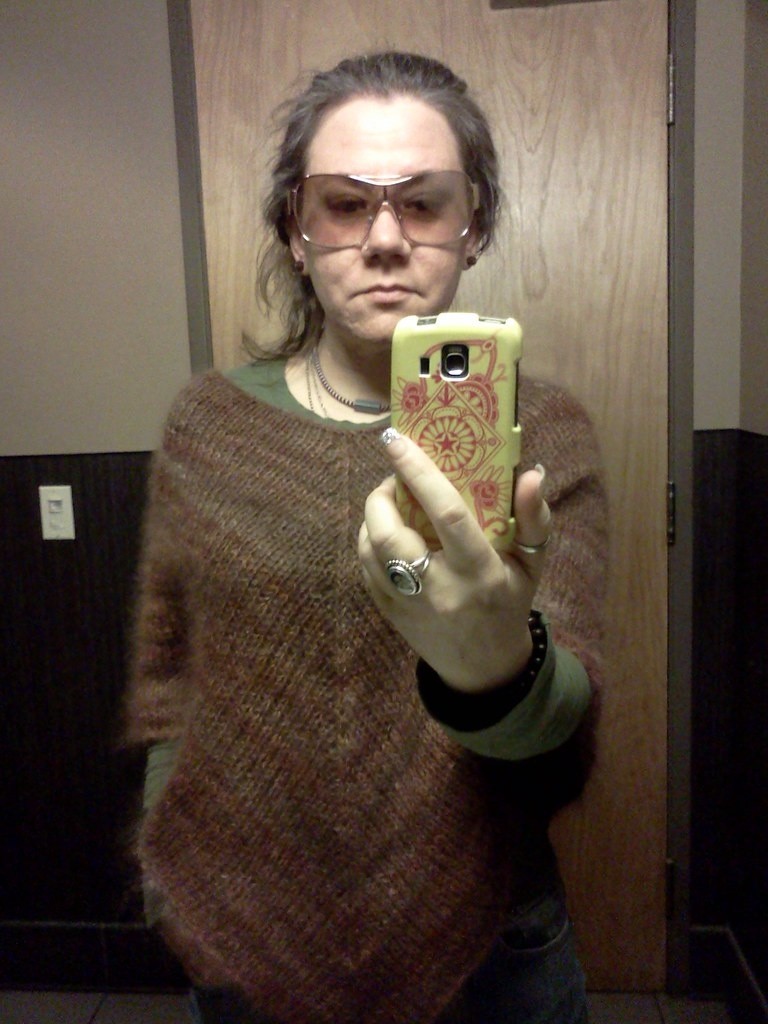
[305,345,391,419]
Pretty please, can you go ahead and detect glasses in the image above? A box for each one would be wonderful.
[287,170,480,249]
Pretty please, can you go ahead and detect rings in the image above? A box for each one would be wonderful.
[385,547,433,598]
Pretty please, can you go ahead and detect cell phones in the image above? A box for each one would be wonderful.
[391,313,523,550]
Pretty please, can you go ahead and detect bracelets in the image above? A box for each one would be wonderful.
[422,610,547,708]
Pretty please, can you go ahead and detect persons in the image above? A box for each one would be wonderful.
[129,50,613,1024]
